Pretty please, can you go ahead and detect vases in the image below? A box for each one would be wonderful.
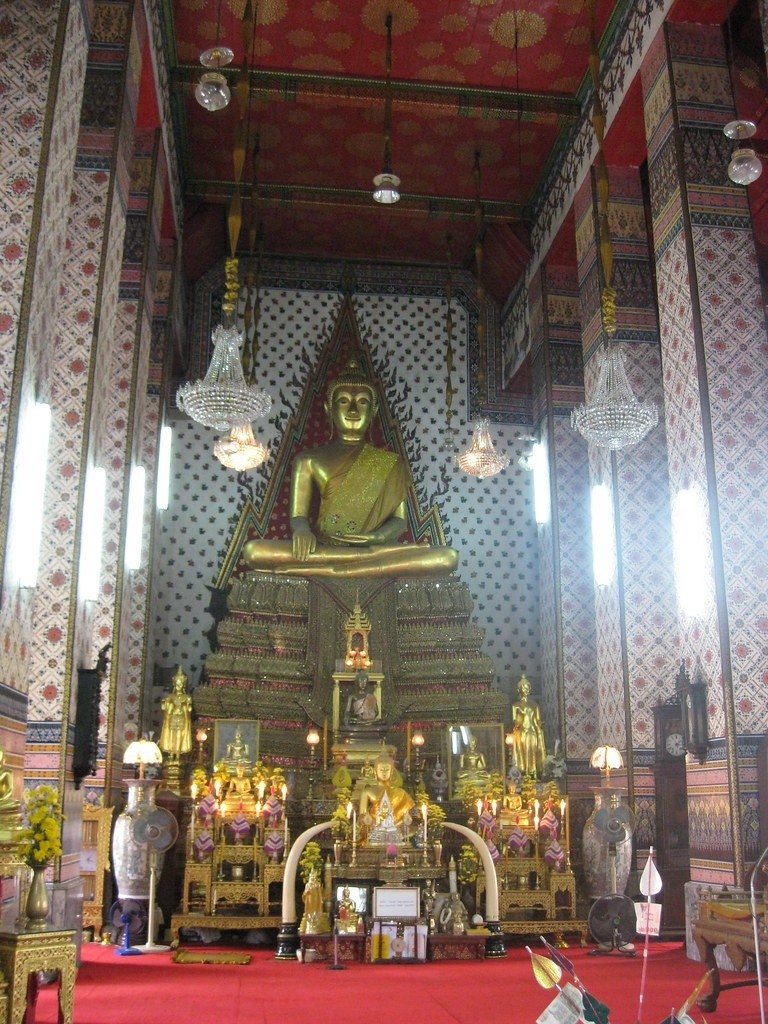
[580,786,634,897]
[331,837,344,866]
[432,840,446,865]
[462,887,474,919]
[27,861,53,929]
[303,877,322,915]
[109,777,168,899]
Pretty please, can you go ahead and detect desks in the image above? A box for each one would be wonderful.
[494,825,540,857]
[494,857,548,890]
[502,890,551,918]
[427,935,489,962]
[298,934,366,965]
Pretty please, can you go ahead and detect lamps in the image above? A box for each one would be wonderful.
[304,727,321,799]
[122,734,163,778]
[454,146,512,482]
[569,0,662,451]
[210,130,271,474]
[587,742,625,788]
[192,0,237,112]
[369,12,405,208]
[194,726,208,768]
[506,730,516,770]
[174,0,277,432]
[411,727,427,794]
[723,20,762,187]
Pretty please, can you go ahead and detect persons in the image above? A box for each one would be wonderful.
[445,892,468,935]
[421,879,436,924]
[486,776,559,826]
[511,673,545,780]
[243,356,458,578]
[160,665,192,763]
[191,724,285,814]
[299,868,332,934]
[0,745,23,811]
[343,670,380,726]
[358,753,413,826]
[455,736,491,795]
[337,884,359,934]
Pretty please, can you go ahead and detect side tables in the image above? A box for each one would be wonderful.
[474,875,502,919]
[545,871,577,920]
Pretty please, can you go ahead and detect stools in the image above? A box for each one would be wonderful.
[0,924,81,1024]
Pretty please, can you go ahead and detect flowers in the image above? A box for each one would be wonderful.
[428,804,448,838]
[11,784,68,863]
[298,839,326,879]
[457,845,478,886]
[330,802,347,837]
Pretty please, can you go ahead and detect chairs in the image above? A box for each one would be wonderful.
[690,879,768,1012]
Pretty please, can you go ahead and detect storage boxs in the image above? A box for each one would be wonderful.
[211,717,262,773]
[447,722,505,800]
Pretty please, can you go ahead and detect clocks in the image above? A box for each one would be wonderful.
[653,692,687,768]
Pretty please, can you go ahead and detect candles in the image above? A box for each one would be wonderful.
[476,796,484,815]
[219,835,225,875]
[211,888,218,907]
[190,778,295,841]
[533,801,540,831]
[492,801,497,815]
[422,806,428,840]
[351,809,359,843]
[559,798,567,817]
[253,834,258,878]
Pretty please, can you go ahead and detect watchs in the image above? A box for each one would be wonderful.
[391,921,406,962]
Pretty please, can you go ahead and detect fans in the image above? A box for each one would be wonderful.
[109,894,147,956]
[593,798,636,893]
[127,803,180,954]
[585,893,641,958]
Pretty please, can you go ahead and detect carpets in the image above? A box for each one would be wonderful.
[170,948,254,965]
